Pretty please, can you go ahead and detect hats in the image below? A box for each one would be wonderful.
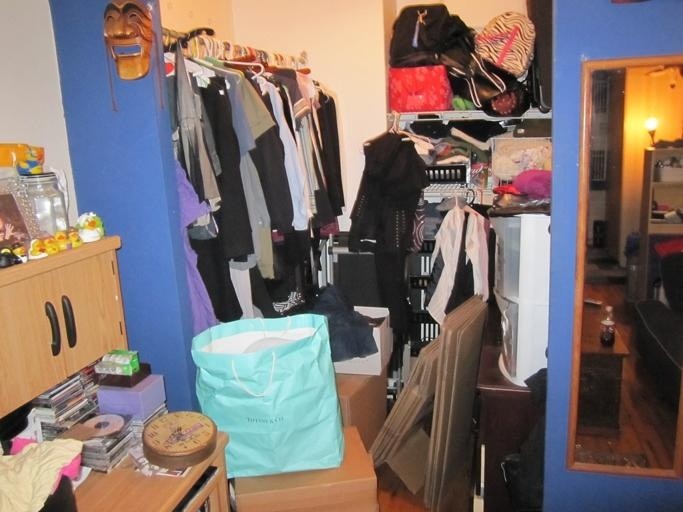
[493,169,551,199]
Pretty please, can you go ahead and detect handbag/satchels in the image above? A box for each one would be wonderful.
[191,315,343,481]
[389,3,536,117]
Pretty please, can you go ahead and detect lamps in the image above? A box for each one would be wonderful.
[645,119,660,145]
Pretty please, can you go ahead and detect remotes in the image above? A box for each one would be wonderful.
[585,296,603,306]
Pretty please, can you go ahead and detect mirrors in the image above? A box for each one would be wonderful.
[565,56,683,478]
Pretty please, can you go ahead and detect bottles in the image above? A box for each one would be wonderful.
[602,306,616,348]
[18,171,71,246]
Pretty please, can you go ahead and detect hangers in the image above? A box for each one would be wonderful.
[435,188,490,224]
[164,32,330,107]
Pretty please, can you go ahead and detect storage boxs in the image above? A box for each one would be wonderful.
[233,425,378,511]
[328,304,396,376]
[388,64,454,113]
[335,372,388,449]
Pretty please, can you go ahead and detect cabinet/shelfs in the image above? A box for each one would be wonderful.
[474,309,544,511]
[574,282,629,439]
[67,429,231,511]
[388,105,553,217]
[637,146,683,303]
[0,234,128,421]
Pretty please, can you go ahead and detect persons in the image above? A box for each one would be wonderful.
[0,218,18,248]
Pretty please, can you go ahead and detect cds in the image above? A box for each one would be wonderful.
[83,414,123,435]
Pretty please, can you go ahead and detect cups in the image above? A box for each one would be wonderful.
[470,163,488,190]
